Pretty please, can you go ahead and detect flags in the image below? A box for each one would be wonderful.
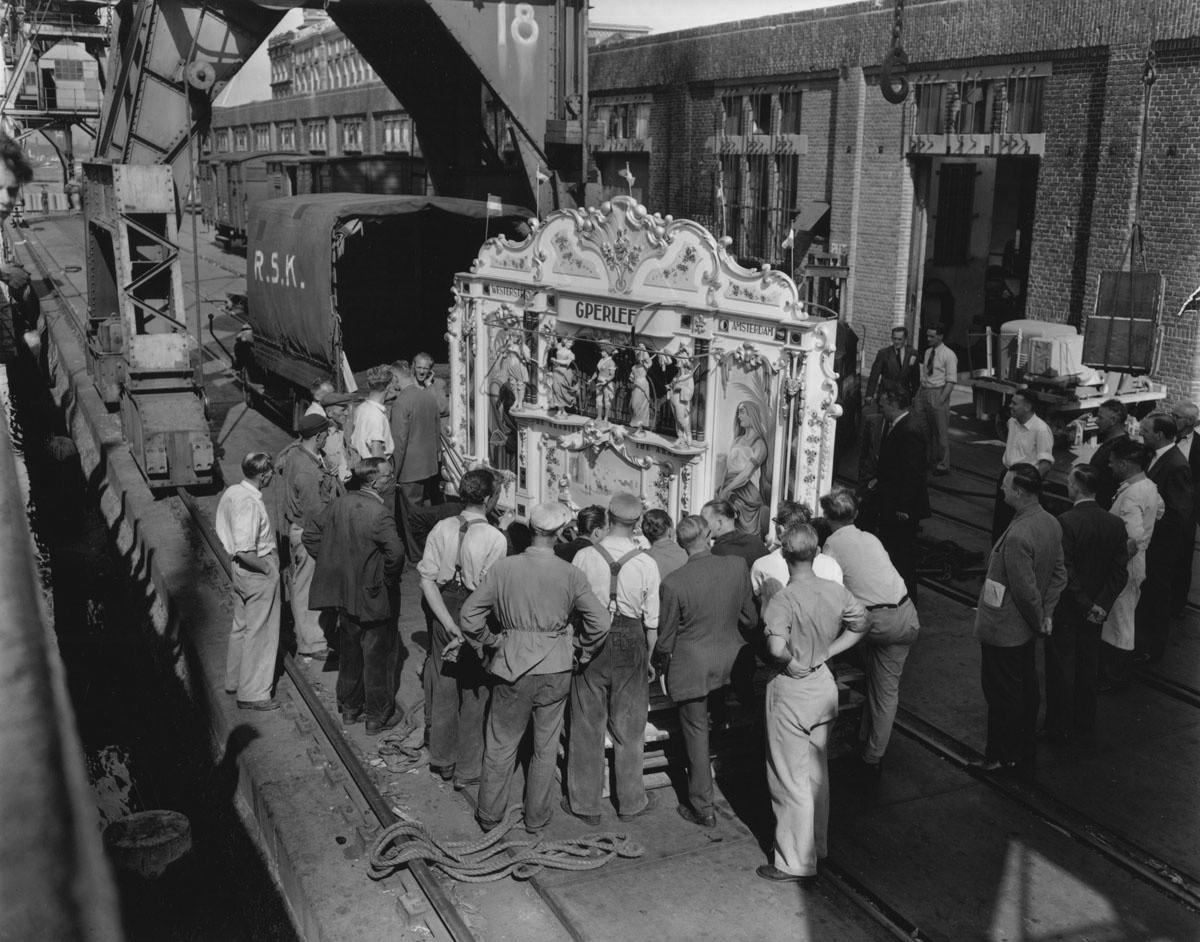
[535,172,550,184]
[486,194,503,217]
[716,187,723,198]
[618,169,635,184]
[782,230,796,250]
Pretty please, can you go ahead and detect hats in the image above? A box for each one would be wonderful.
[298,414,330,436]
[608,490,643,525]
[529,500,572,533]
[322,392,353,406]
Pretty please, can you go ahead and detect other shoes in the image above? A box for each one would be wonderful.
[848,760,882,780]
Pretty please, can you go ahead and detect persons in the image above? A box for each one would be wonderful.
[815,490,922,763]
[1082,399,1138,469]
[1091,441,1166,693]
[858,379,933,599]
[1136,412,1197,648]
[920,322,961,476]
[963,463,1071,779]
[291,346,878,888]
[869,326,921,442]
[987,390,1056,546]
[215,450,290,711]
[1,131,31,314]
[1039,463,1131,744]
[1170,400,1196,620]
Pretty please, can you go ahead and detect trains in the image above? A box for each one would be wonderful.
[200,150,428,262]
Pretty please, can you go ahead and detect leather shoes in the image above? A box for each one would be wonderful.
[759,864,818,885]
[965,757,1005,772]
[528,808,554,833]
[561,796,601,824]
[475,812,499,830]
[238,699,283,711]
[1002,759,1015,768]
[367,712,402,734]
[621,790,658,821]
[226,688,236,695]
[437,762,455,778]
[452,776,481,788]
[342,711,360,725]
[677,803,716,828]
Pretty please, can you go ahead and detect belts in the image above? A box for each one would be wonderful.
[775,662,824,677]
[866,593,910,611]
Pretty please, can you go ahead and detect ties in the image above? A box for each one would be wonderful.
[927,348,935,375]
[1174,436,1188,444]
[1144,450,1156,473]
[896,347,901,368]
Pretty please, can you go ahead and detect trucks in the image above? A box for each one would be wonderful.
[234,190,536,437]
